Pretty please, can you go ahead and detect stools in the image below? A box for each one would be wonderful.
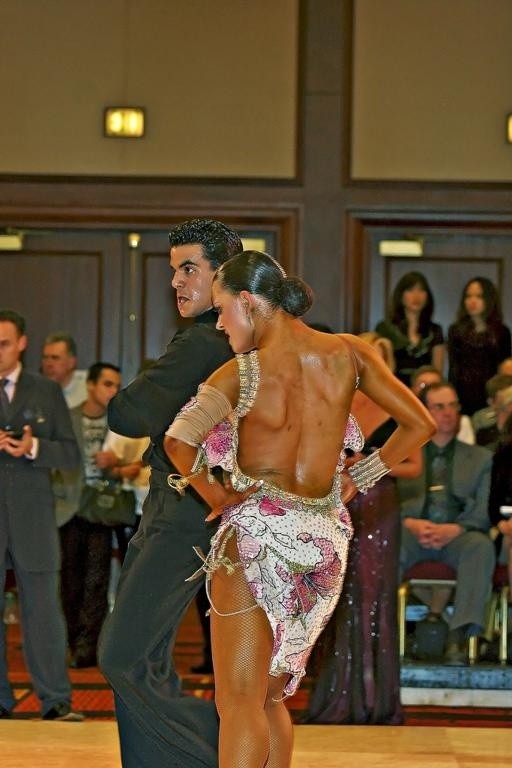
[397,559,511,668]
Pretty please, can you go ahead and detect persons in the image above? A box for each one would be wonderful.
[1,310,86,720]
[302,322,424,726]
[42,331,212,673]
[374,272,512,665]
[97,217,244,767]
[163,250,437,766]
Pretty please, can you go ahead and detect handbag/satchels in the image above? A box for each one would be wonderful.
[77,485,135,526]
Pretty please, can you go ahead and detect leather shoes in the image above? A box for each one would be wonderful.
[191,662,213,674]
[43,704,84,720]
[443,639,469,666]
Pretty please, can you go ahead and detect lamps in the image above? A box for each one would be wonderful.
[104,105,147,138]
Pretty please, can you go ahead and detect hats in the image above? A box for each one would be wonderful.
[485,358,512,398]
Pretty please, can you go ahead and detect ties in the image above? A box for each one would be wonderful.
[0,378,10,410]
